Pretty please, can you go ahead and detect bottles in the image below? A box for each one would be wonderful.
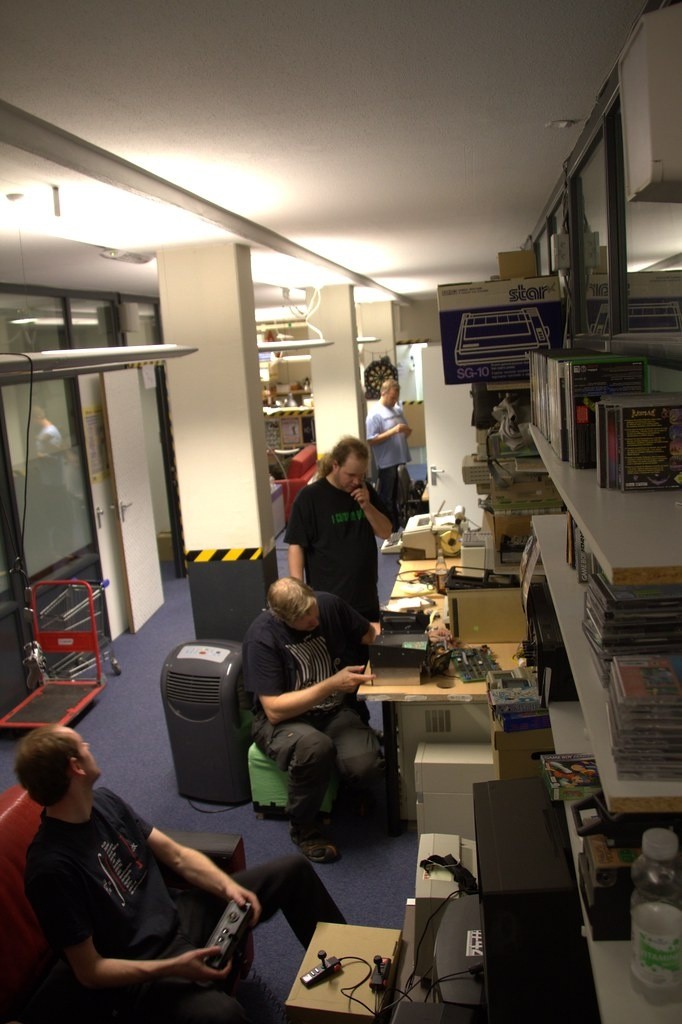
[628,827,682,1007]
[435,548,448,595]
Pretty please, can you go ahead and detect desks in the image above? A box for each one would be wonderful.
[356,557,532,706]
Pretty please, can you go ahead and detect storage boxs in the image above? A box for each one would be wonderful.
[565,271,681,363]
[436,275,566,386]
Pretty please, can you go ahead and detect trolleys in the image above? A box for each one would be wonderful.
[0,577,106,733]
[24,579,124,681]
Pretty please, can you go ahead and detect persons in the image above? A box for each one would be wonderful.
[15,726,348,1024]
[366,380,412,548]
[243,576,452,863]
[30,406,80,560]
[284,438,394,743]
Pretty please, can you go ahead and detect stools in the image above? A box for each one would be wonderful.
[248,740,335,819]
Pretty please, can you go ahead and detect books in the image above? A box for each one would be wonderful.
[539,752,601,800]
[527,350,682,782]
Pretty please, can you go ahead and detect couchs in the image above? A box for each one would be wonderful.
[275,443,320,527]
[0,785,255,1023]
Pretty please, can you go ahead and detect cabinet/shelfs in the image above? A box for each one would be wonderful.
[462,133,682,1024]
